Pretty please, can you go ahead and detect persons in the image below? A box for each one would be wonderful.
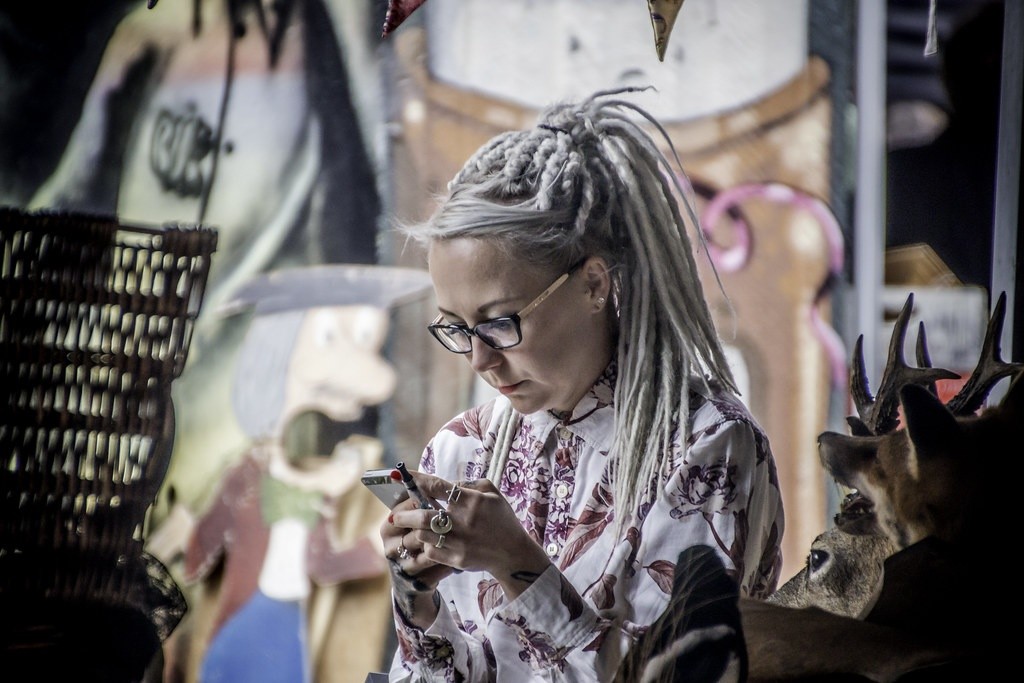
[379,79,788,683]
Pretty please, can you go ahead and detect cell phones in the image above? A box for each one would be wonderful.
[361,469,411,510]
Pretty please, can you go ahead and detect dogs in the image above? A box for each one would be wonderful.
[814,371,1023,552]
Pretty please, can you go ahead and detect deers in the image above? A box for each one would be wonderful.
[763,286,1024,620]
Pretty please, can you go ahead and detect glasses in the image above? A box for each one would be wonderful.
[428,255,588,354]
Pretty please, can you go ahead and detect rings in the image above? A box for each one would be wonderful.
[397,533,418,563]
[435,535,445,549]
[445,483,462,503]
[430,508,453,534]
